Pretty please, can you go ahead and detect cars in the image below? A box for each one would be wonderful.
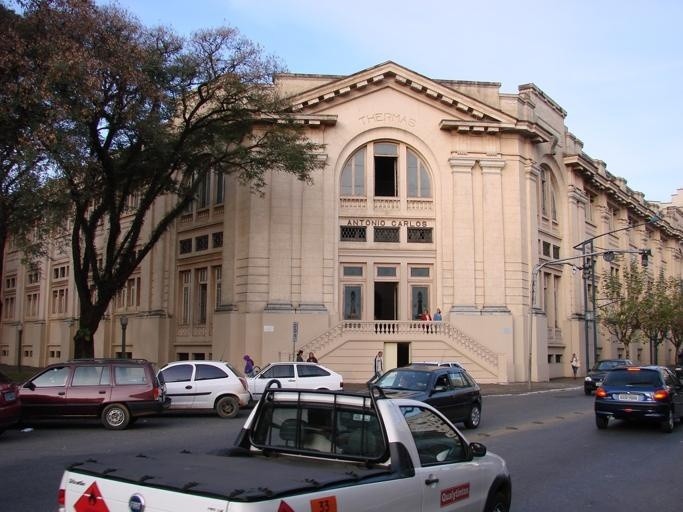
[157,360,249,419]
[245,361,344,403]
[0,373,20,425]
[584,358,632,394]
[402,361,462,368]
[354,366,481,429]
[13,359,170,429]
[593,367,681,429]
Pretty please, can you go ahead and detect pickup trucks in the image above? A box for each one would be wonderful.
[55,381,512,512]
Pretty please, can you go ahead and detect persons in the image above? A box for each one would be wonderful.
[366,351,383,388]
[297,350,304,363]
[307,351,318,362]
[419,309,433,334]
[300,409,351,454]
[396,374,410,387]
[243,353,255,374]
[571,352,579,380]
[433,308,442,334]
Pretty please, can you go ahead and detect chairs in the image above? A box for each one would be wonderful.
[277,417,312,449]
[342,427,377,457]
